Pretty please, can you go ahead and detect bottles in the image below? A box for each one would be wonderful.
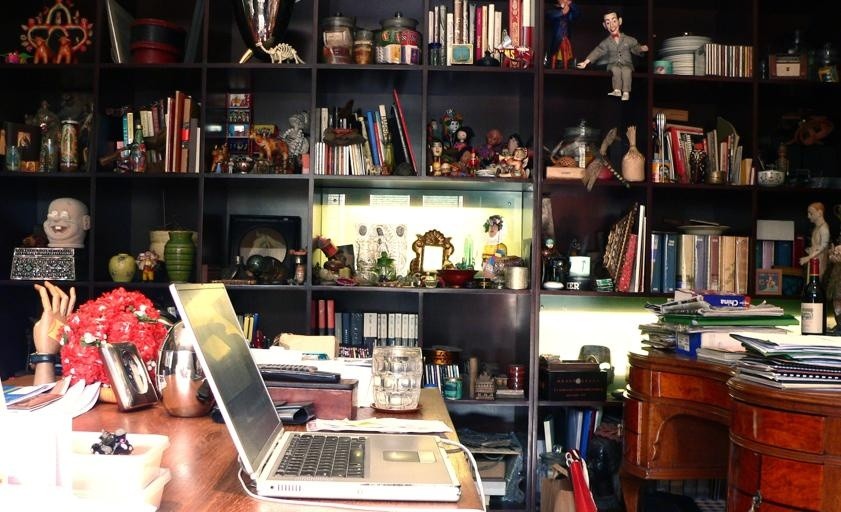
[785,25,841,83]
[801,258,825,334]
[288,248,307,285]
[130,124,148,174]
[621,126,646,183]
[5,98,81,173]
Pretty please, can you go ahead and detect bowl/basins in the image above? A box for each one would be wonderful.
[437,269,479,289]
[756,170,786,186]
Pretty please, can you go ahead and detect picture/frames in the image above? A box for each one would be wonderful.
[98,341,161,413]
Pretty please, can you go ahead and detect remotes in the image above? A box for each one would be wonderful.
[257,363,316,370]
[261,369,339,383]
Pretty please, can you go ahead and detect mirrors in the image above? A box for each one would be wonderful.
[410,230,453,274]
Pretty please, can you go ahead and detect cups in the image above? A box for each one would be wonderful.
[443,377,463,400]
[352,29,375,65]
[503,266,528,291]
[506,363,527,389]
[373,347,422,412]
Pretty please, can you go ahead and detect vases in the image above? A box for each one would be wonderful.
[109,254,136,282]
[165,231,194,283]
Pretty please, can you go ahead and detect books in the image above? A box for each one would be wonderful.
[428,0,532,68]
[310,297,418,348]
[315,90,419,178]
[1,376,102,420]
[122,90,201,172]
[639,109,841,393]
[693,43,753,78]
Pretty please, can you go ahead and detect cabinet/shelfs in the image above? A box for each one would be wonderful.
[530,0,841,512]
[0,0,539,511]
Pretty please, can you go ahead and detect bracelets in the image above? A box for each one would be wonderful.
[30,351,56,363]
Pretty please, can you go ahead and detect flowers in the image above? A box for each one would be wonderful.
[58,288,165,404]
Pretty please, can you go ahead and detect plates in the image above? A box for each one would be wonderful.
[657,35,713,76]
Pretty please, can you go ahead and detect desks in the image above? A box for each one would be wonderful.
[0,375,483,512]
[619,352,840,511]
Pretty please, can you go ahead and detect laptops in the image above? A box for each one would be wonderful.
[171,283,462,502]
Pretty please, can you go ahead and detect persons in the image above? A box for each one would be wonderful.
[576,11,648,101]
[30,280,77,386]
[548,0,575,71]
[41,197,90,251]
[426,106,531,179]
[20,1,93,64]
[136,250,157,281]
[480,214,505,272]
